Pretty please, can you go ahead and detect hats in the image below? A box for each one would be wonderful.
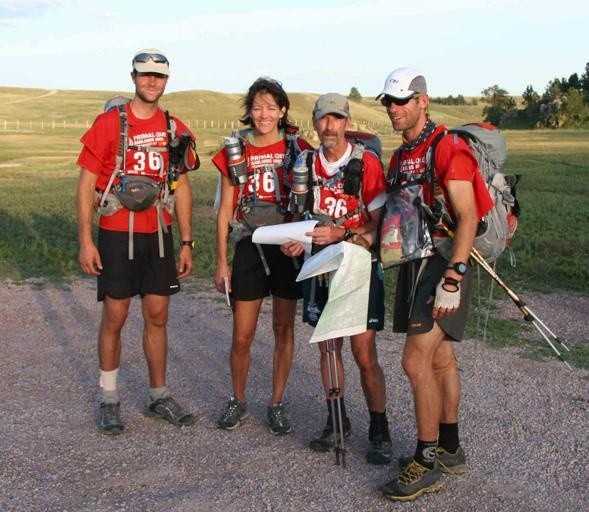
[375,69,427,101]
[313,93,349,121]
[132,49,169,78]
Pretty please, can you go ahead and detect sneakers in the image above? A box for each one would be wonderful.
[366,425,393,464]
[399,444,466,474]
[310,418,351,452]
[379,457,443,501]
[98,402,125,435]
[217,396,248,430]
[265,401,292,435]
[147,394,195,426]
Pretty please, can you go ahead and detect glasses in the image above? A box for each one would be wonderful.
[381,93,423,108]
[132,53,169,68]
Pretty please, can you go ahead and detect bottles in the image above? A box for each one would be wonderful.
[223,142,248,184]
[290,164,309,206]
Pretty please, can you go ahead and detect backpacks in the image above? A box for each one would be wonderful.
[305,131,382,226]
[395,122,519,266]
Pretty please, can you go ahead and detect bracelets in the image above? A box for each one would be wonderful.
[343,227,353,241]
[180,238,198,251]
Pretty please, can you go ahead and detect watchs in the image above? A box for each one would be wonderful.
[439,260,469,277]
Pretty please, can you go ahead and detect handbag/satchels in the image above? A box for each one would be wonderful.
[112,177,161,211]
[240,201,286,232]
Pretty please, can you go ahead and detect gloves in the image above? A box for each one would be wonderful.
[429,275,461,310]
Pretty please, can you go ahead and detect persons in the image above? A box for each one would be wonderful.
[373,66,496,503]
[73,45,201,437]
[280,92,394,467]
[210,74,318,440]
